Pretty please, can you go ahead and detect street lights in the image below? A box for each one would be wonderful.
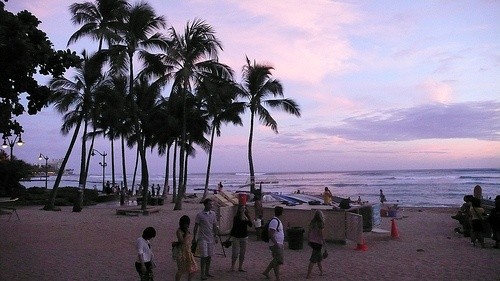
[38,154,50,188]
[92,148,109,192]
[2,132,23,162]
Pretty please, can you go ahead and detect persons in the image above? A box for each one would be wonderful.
[255,196,263,241]
[262,206,285,281]
[194,198,219,281]
[324,187,332,205]
[458,195,500,249]
[171,215,198,281]
[226,204,253,272]
[135,227,156,281]
[305,210,328,278]
[339,189,400,217]
[93,180,223,198]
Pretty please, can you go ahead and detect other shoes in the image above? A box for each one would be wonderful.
[471,241,500,249]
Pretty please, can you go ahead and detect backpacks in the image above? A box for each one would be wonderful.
[262,223,269,243]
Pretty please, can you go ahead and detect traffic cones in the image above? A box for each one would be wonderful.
[390,219,400,237]
[356,239,369,251]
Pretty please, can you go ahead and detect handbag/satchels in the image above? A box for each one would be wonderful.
[191,240,197,253]
[171,241,181,258]
[481,220,491,233]
[151,260,156,272]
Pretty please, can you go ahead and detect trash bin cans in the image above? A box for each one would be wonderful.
[287,225,305,250]
